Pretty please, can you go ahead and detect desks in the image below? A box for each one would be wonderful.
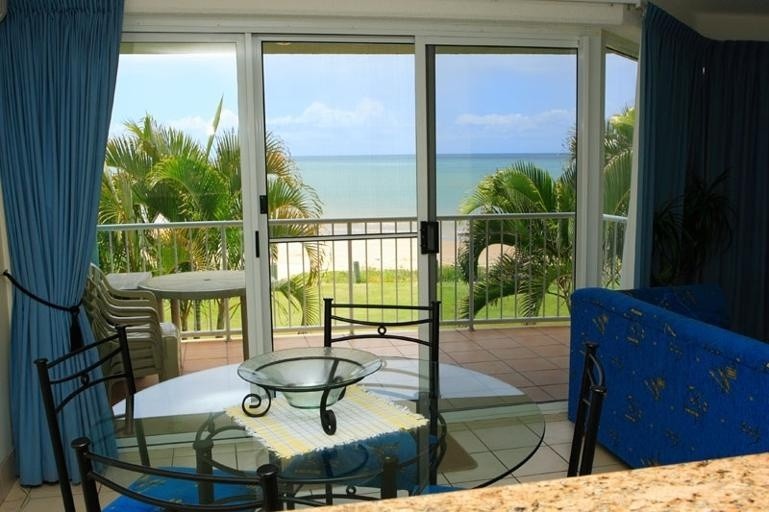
[137,268,282,365]
[70,351,549,512]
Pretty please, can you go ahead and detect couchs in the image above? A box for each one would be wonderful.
[555,277,768,477]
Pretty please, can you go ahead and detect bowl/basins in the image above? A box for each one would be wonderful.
[237,346,382,408]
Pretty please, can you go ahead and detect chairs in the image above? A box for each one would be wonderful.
[408,337,611,500]
[83,261,184,406]
[281,297,448,501]
[69,435,285,512]
[33,325,263,512]
